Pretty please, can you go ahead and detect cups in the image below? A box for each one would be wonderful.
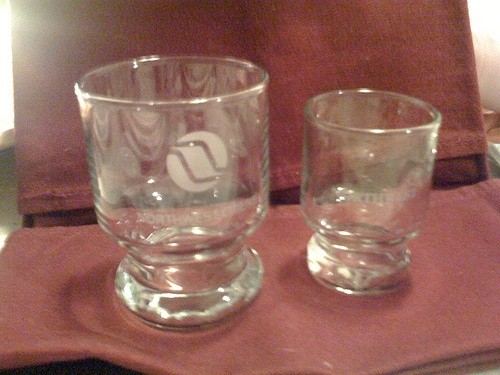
[73,53,272,333]
[299,89,441,295]
